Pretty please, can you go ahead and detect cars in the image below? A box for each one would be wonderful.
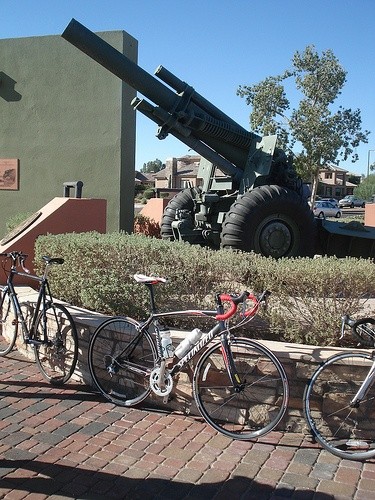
[308,201,343,219]
[339,195,366,208]
[319,198,339,206]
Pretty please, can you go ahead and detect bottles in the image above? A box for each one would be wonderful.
[175,328,202,358]
[160,326,173,359]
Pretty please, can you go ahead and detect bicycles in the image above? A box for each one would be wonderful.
[0,250,79,383]
[301,314,375,464]
[86,273,293,440]
